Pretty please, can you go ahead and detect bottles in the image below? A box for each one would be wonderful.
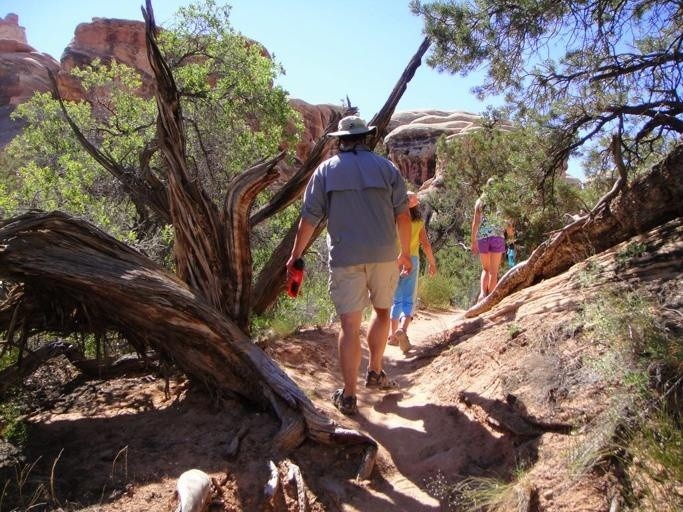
[507,243,515,268]
[287,257,305,299]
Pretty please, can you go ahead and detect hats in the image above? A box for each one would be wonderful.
[406,190,419,208]
[327,116,378,138]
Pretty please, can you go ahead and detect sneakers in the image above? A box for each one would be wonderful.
[387,329,411,351]
[333,388,357,415]
[364,367,401,390]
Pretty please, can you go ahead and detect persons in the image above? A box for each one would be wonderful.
[471,178,517,304]
[388,190,436,352]
[287,115,412,415]
